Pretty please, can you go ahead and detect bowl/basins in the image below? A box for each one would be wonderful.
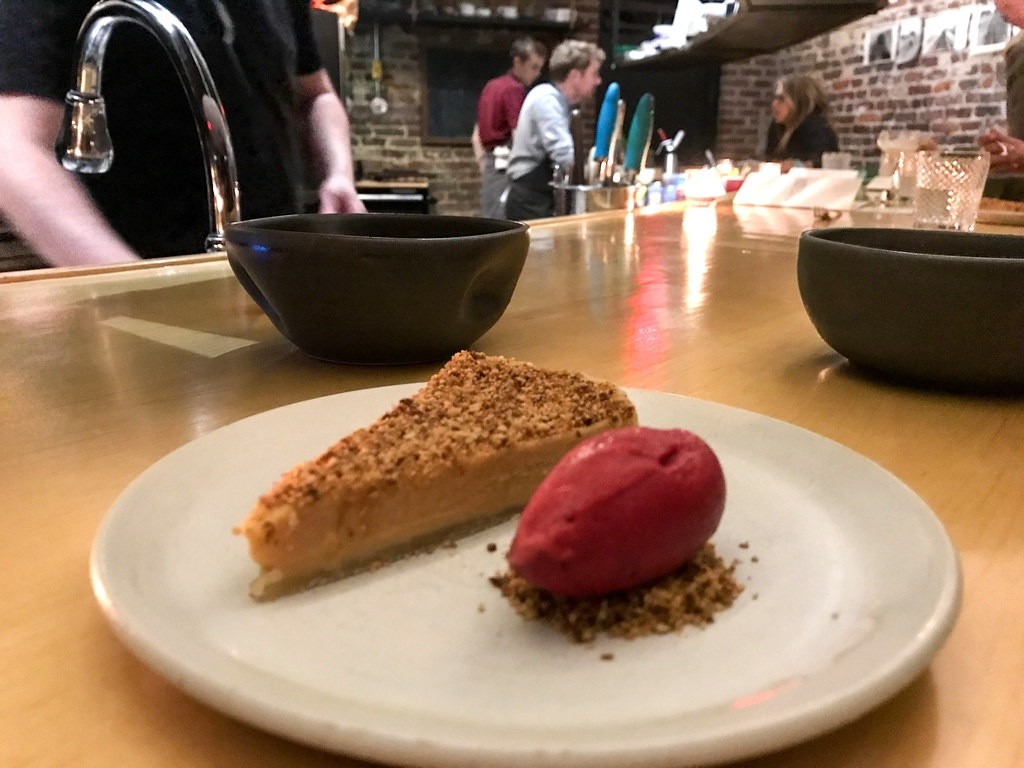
[796,226,1023,390]
[224,214,530,366]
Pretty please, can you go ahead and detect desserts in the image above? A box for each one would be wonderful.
[242,350,742,643]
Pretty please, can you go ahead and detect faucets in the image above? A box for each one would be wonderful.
[51,1,248,255]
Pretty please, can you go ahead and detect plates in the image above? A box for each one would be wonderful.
[91,373,964,766]
[976,196,1024,226]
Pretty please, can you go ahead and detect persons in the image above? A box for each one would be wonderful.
[500,38,606,221]
[470,40,547,220]
[978,0,1023,201]
[766,74,839,173]
[1,1,371,268]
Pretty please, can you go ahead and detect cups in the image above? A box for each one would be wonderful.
[822,152,852,170]
[911,148,990,233]
[550,176,639,220]
[451,3,518,19]
[898,150,918,200]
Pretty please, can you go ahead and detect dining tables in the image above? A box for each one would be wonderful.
[0,193,1024,768]
